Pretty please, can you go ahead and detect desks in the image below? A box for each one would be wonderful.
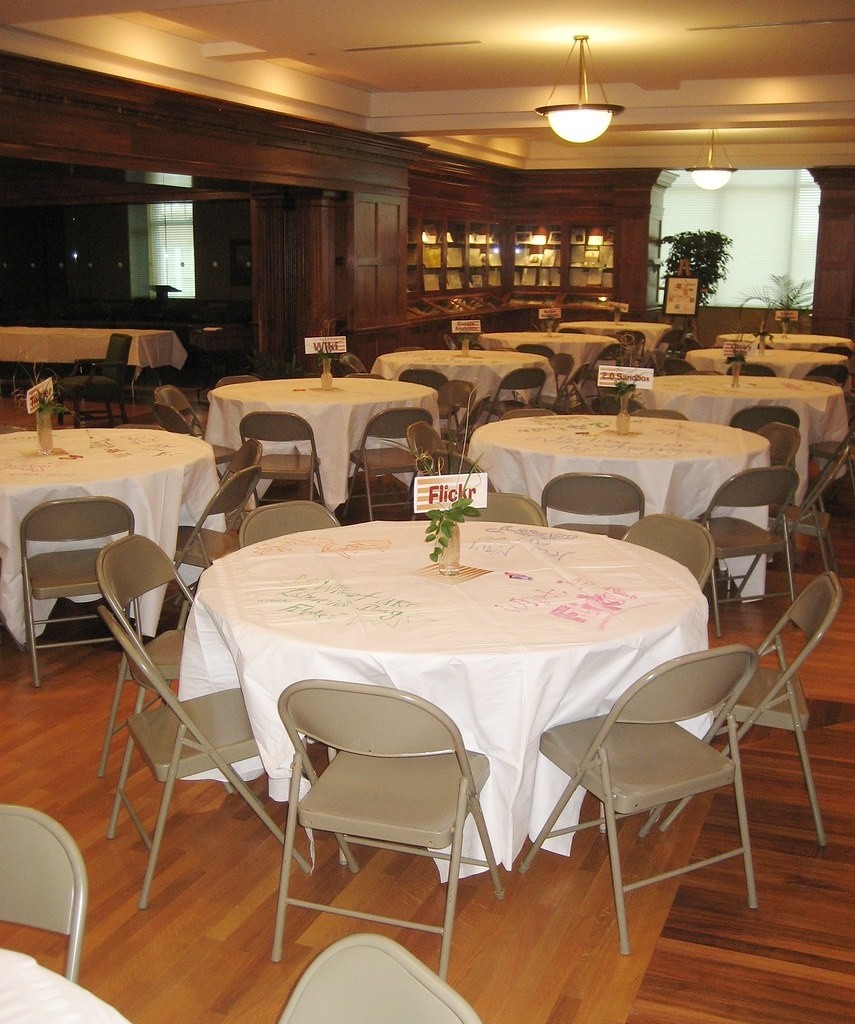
[174,520,714,886]
[627,375,850,508]
[478,333,621,407]
[203,378,439,512]
[1,428,227,652]
[554,320,672,354]
[714,333,855,354]
[467,414,772,605]
[370,350,556,434]
[1,325,189,398]
[685,348,853,394]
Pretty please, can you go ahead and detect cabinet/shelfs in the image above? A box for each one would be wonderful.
[407,203,619,299]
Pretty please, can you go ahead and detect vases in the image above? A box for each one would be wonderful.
[782,321,788,338]
[546,321,553,337]
[321,357,333,390]
[616,395,631,435]
[759,335,766,354]
[731,361,742,387]
[461,338,469,357]
[37,411,52,455]
[615,310,619,324]
[439,521,461,575]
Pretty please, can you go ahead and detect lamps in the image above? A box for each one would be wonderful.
[534,34,625,143]
[686,128,738,191]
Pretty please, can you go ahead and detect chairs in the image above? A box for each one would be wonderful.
[0,311,855,1023]
